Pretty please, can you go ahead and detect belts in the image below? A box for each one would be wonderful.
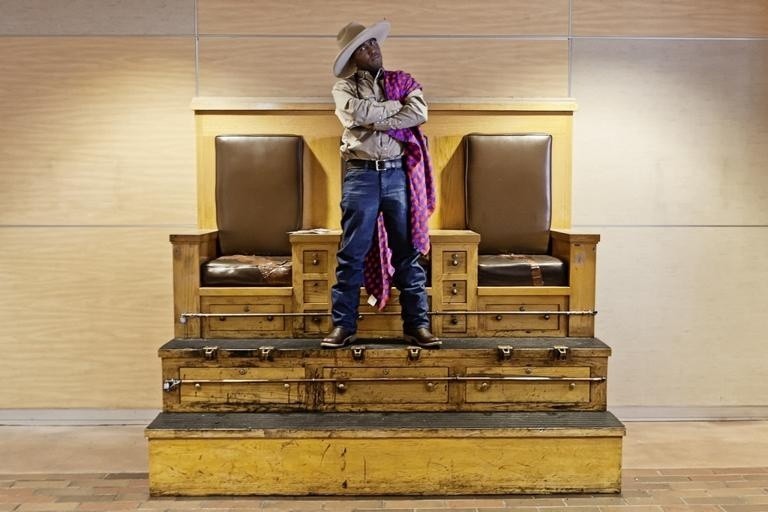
[344,160,404,171]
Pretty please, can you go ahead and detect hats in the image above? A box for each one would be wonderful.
[332,19,391,78]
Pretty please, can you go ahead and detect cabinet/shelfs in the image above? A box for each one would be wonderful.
[286,229,482,336]
[199,294,290,337]
[479,296,566,338]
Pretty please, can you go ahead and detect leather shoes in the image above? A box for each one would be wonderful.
[402,327,442,346]
[320,326,356,348]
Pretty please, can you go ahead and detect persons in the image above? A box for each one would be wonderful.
[320,19,443,348]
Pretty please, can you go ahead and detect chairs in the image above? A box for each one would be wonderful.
[170,133,305,298]
[459,134,600,292]
